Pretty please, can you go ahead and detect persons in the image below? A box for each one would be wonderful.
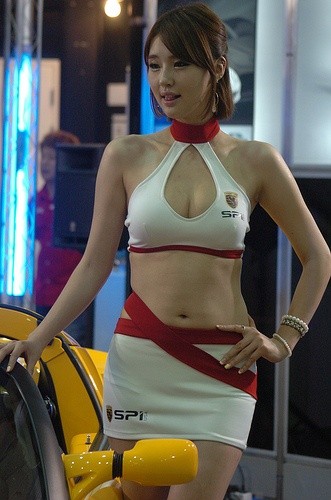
[33,131,94,346]
[0,3,331,500]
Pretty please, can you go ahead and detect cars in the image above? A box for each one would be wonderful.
[0,303,198,498]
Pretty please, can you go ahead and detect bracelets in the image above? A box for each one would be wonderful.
[272,333,292,356]
[279,314,309,336]
